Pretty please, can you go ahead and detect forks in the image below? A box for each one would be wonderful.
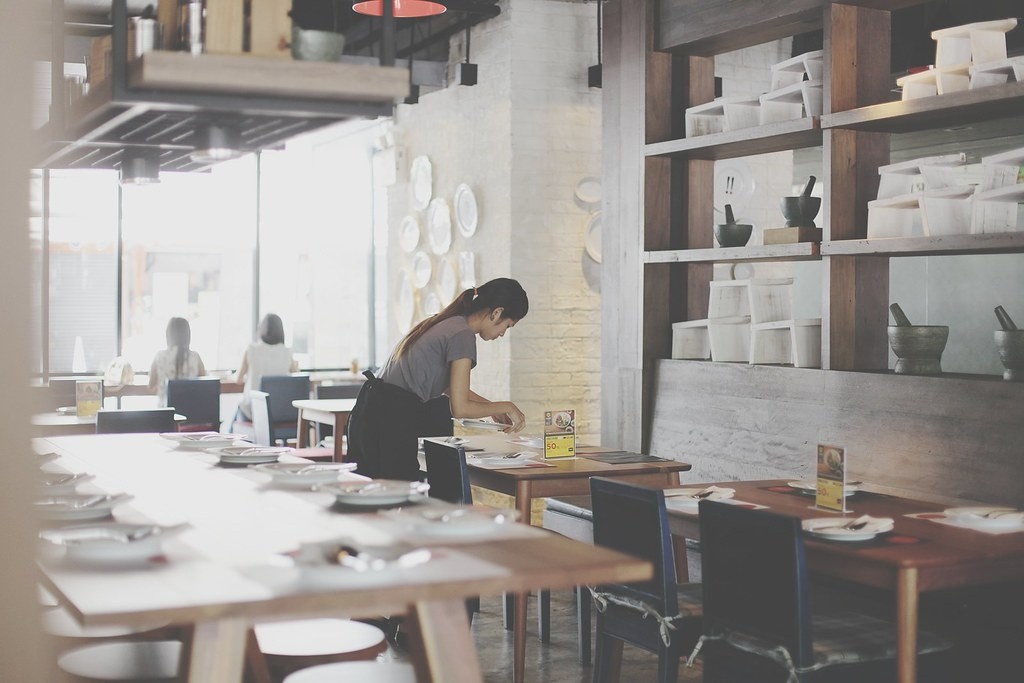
[810,518,858,531]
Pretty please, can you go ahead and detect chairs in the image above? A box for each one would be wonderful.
[96,407,175,433]
[166,379,221,433]
[424,439,957,683]
[315,385,362,440]
[261,376,310,446]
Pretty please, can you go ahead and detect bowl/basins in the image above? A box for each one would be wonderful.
[713,224,753,248]
[292,28,345,61]
[823,450,842,471]
[553,411,575,432]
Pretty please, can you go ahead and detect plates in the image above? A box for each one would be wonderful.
[584,210,601,264]
[443,437,470,445]
[487,454,526,465]
[943,504,1024,529]
[31,435,428,568]
[787,479,858,497]
[663,487,736,506]
[396,155,478,311]
[451,417,512,430]
[800,516,895,542]
[575,175,603,203]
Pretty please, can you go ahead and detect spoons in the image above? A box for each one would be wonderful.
[664,491,714,499]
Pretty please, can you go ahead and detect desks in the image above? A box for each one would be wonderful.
[292,398,357,462]
[468,444,692,683]
[31,434,653,683]
[662,478,1024,683]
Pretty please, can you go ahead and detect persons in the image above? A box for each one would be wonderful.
[149,317,204,409]
[228,313,299,433]
[347,277,529,645]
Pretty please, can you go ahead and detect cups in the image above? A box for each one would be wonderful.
[128,17,163,61]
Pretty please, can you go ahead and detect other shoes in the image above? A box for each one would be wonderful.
[394,631,409,649]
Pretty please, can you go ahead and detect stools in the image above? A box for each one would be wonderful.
[46,610,415,683]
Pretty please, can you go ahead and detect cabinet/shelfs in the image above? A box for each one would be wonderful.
[644,81,1024,263]
